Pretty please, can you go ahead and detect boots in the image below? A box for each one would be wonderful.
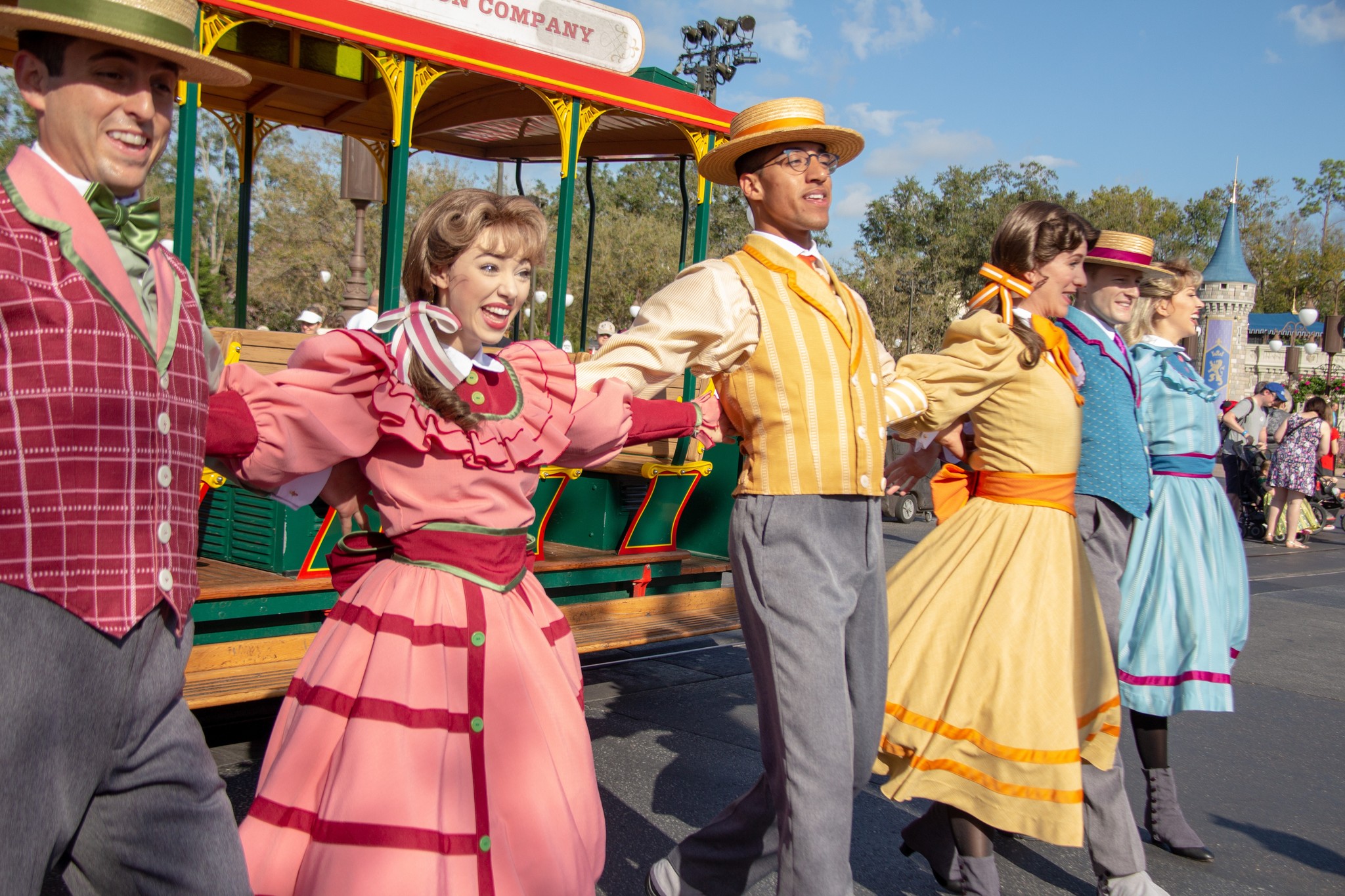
[1142,766,1218,862]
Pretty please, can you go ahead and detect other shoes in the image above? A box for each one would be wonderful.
[646,860,704,896]
[1096,868,1172,896]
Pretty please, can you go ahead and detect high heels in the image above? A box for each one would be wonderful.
[900,803,1004,895]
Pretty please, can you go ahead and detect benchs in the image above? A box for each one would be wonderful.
[208,323,718,573]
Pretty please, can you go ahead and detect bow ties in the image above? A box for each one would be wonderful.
[83,180,162,251]
[1029,314,1079,379]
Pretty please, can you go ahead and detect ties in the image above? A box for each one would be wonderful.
[799,254,834,297]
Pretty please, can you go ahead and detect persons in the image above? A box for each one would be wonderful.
[295,303,328,336]
[201,183,738,896]
[345,287,382,334]
[0,0,259,896]
[855,197,1126,896]
[1113,255,1256,865]
[1217,376,1345,552]
[573,94,977,896]
[588,319,617,357]
[880,223,1181,896]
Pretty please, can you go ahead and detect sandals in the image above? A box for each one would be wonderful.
[1263,534,1274,544]
[1285,540,1310,548]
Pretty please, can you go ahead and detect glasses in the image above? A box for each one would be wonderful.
[750,148,837,178]
[1270,392,1281,402]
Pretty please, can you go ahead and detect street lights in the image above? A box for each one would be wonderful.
[1267,320,1317,392]
[1304,277,1345,395]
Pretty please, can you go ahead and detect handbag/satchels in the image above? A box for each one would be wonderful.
[1263,488,1321,538]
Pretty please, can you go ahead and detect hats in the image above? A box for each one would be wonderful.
[1265,382,1287,403]
[699,98,866,185]
[1084,229,1177,279]
[0,0,254,90]
